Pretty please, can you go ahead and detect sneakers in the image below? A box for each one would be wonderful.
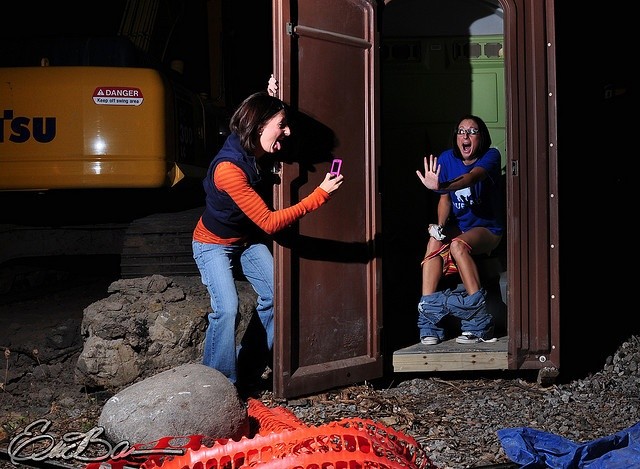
[232,383,262,407]
[421,335,440,345]
[456,331,498,344]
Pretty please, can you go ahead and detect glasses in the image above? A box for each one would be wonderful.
[456,129,480,135]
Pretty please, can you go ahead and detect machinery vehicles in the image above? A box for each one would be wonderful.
[0,34,218,303]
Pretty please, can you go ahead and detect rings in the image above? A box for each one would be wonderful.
[433,169,435,171]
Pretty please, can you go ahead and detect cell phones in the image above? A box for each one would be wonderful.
[330,159,343,179]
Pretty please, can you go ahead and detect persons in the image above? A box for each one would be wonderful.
[416,114,507,346]
[191,74,344,390]
